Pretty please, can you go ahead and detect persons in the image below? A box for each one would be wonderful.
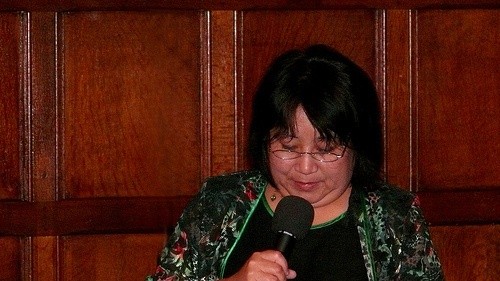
[144,43,446,281]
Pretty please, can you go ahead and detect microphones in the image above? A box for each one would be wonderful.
[271,195,314,260]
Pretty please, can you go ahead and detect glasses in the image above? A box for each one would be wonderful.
[268,136,345,162]
